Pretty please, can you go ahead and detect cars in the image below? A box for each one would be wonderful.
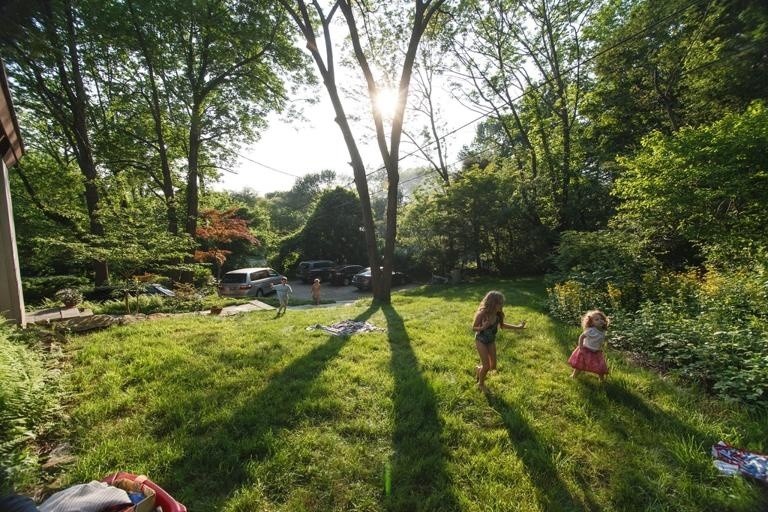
[327,264,410,289]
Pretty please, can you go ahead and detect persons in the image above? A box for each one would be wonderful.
[566,311,610,382]
[270,277,293,315]
[310,278,321,305]
[471,292,528,395]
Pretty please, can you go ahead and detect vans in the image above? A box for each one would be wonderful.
[217,267,287,297]
[296,259,339,282]
